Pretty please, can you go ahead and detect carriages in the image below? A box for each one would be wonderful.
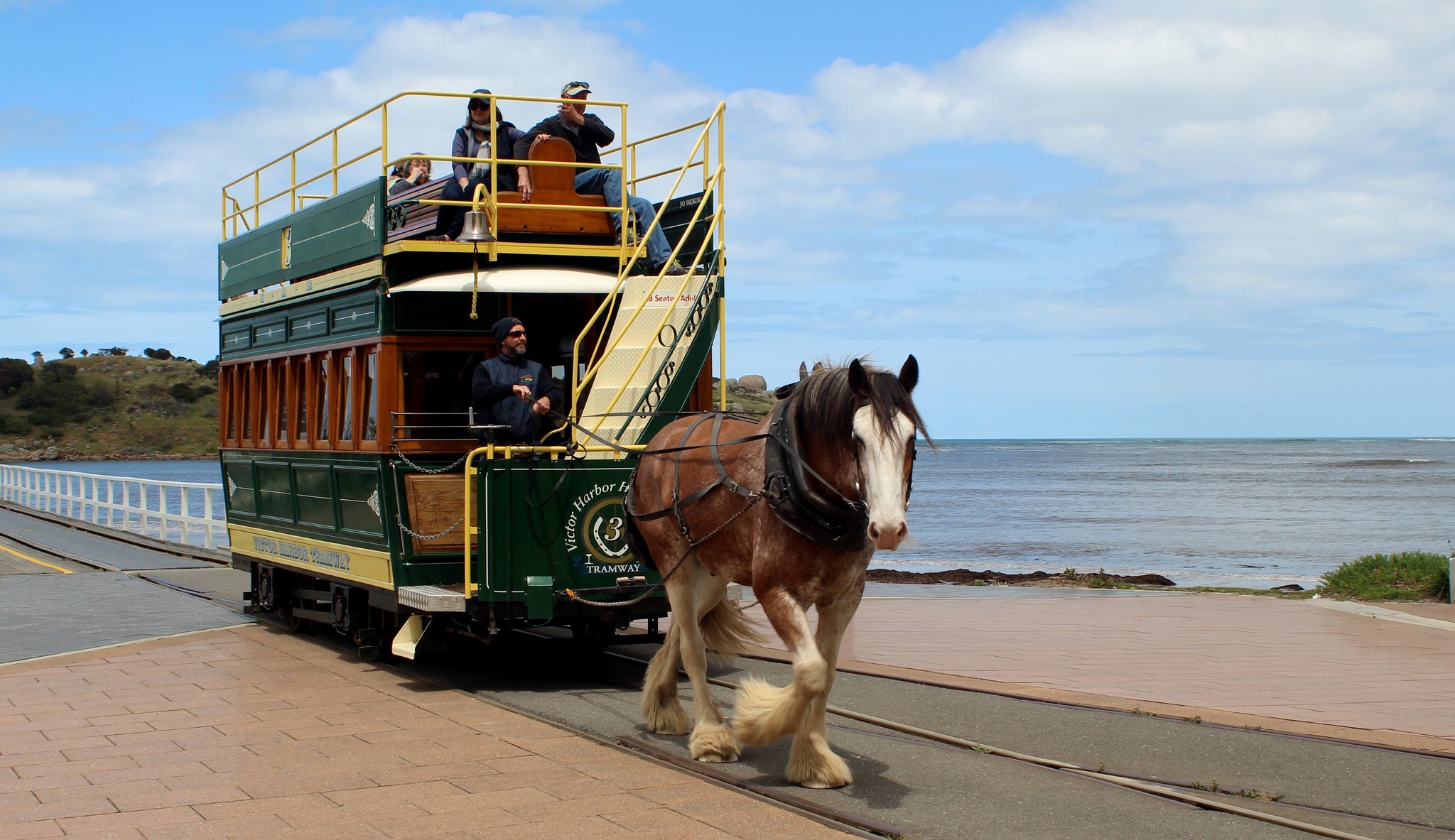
[218,91,940,789]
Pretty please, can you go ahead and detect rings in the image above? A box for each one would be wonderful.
[418,170,420,172]
[566,106,568,109]
[520,189,522,192]
[539,410,540,412]
[541,411,543,413]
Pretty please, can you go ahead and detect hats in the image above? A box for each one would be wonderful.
[471,89,492,104]
[492,318,524,346]
[561,82,592,95]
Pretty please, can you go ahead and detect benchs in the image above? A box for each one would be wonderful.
[385,136,616,245]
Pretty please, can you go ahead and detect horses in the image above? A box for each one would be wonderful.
[626,352,937,789]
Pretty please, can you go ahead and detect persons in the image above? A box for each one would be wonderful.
[387,152,433,198]
[425,89,551,240]
[513,81,696,275]
[472,318,564,460]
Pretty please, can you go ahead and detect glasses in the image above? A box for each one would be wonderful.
[409,163,429,169]
[563,81,589,94]
[506,331,526,337]
[469,102,490,111]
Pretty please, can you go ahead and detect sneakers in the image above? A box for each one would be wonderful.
[665,258,696,276]
[614,227,647,246]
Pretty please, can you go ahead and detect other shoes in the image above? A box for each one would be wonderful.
[423,233,446,241]
